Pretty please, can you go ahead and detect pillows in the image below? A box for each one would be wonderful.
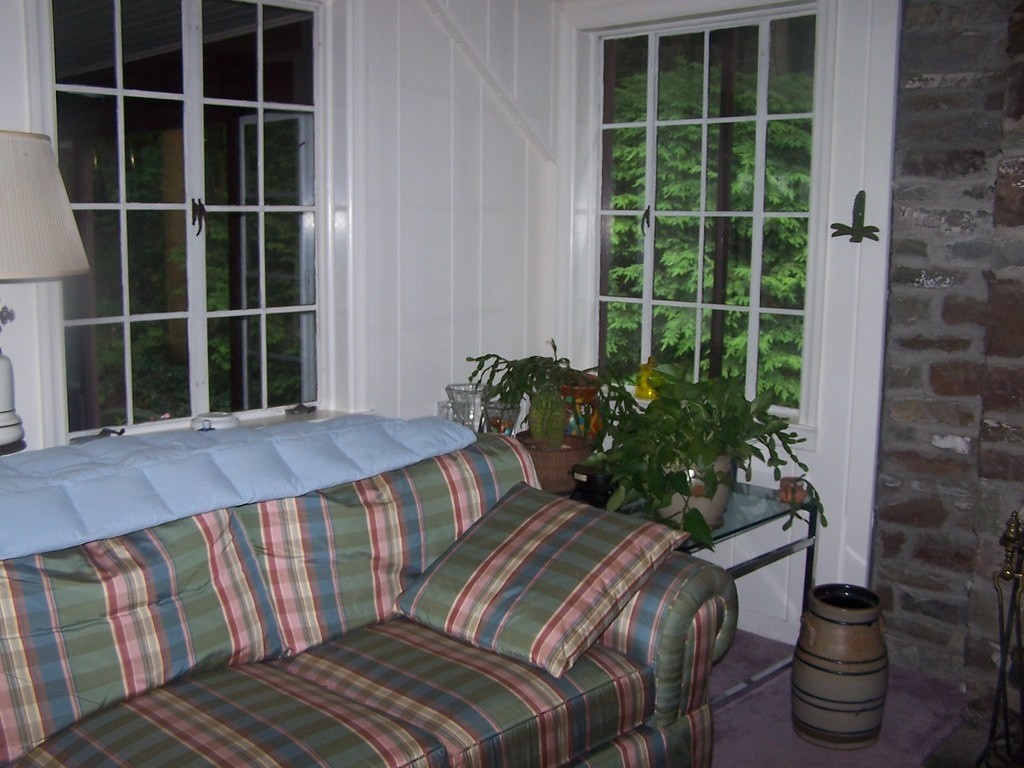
[388,480,691,679]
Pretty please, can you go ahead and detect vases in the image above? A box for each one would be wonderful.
[438,381,521,439]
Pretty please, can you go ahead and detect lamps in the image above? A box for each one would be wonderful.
[0,130,91,451]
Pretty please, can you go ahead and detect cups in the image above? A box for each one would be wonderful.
[483,402,520,438]
[445,384,490,433]
[437,401,464,427]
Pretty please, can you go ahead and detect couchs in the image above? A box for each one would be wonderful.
[0,413,740,768]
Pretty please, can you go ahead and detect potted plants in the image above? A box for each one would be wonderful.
[780,473,828,530]
[469,335,642,459]
[520,381,593,491]
[574,369,810,553]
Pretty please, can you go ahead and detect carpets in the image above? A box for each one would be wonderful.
[707,627,991,768]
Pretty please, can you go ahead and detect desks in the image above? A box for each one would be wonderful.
[675,482,819,714]
